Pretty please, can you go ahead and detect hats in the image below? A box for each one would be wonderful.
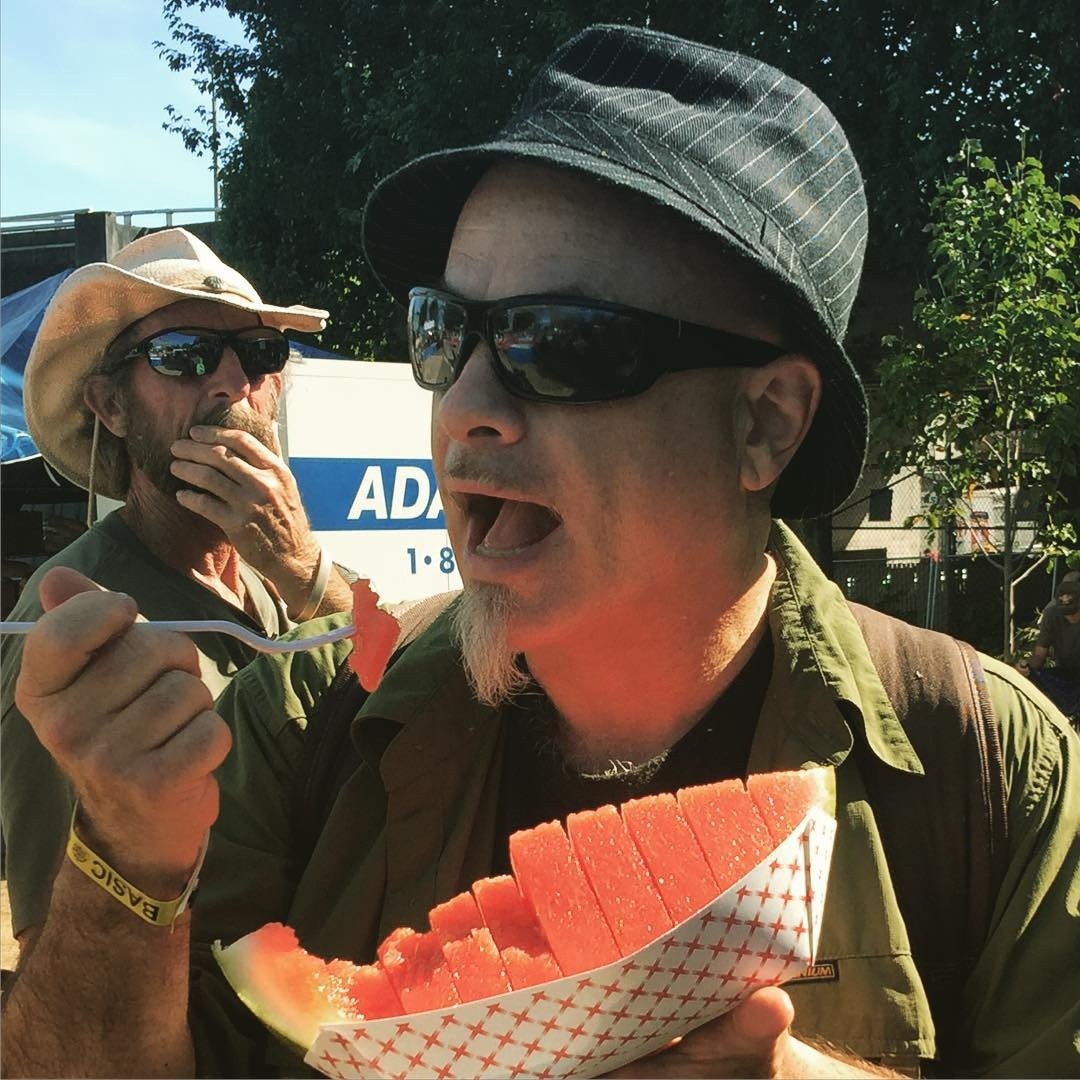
[362,23,872,524]
[23,228,330,503]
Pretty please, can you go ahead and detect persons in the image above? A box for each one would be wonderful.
[1,230,364,975]
[1,20,1080,1080]
[1016,570,1080,715]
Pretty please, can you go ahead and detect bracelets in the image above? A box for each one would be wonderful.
[288,548,334,625]
[66,794,211,934]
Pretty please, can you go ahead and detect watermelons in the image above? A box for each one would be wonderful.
[212,762,837,1051]
[347,578,402,693]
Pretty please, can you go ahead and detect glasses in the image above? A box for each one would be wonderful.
[100,326,290,377]
[405,287,799,406]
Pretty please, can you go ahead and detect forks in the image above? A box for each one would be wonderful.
[0,618,358,656]
